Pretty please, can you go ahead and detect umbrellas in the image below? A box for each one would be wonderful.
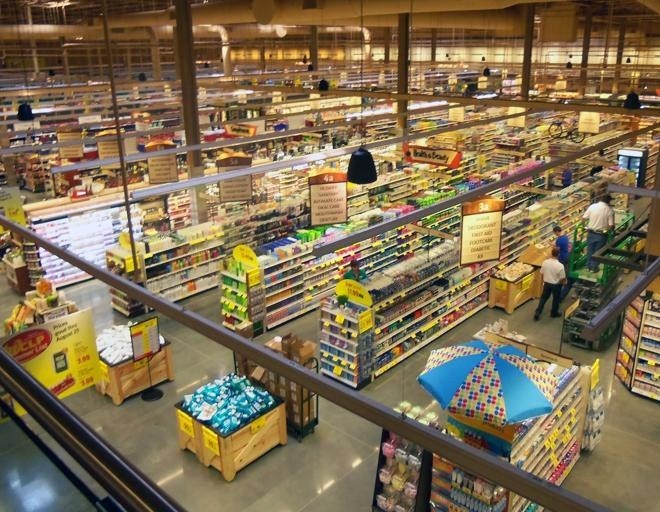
[417,340,559,428]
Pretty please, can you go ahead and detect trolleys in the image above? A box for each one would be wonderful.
[233,349,318,443]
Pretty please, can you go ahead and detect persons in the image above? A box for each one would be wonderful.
[344,260,368,282]
[535,226,568,320]
[582,195,615,273]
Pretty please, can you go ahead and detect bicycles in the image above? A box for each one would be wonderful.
[548,115,585,143]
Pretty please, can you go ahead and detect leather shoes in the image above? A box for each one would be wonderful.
[534,311,562,320]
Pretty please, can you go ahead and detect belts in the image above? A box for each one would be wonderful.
[545,282,560,286]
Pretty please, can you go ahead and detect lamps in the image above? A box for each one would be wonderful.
[346,0,377,184]
[623,1,646,110]
[11,0,34,120]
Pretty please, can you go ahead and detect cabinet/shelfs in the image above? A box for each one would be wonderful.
[1,70,658,417]
[176,374,287,483]
[246,335,320,442]
[372,321,608,511]
